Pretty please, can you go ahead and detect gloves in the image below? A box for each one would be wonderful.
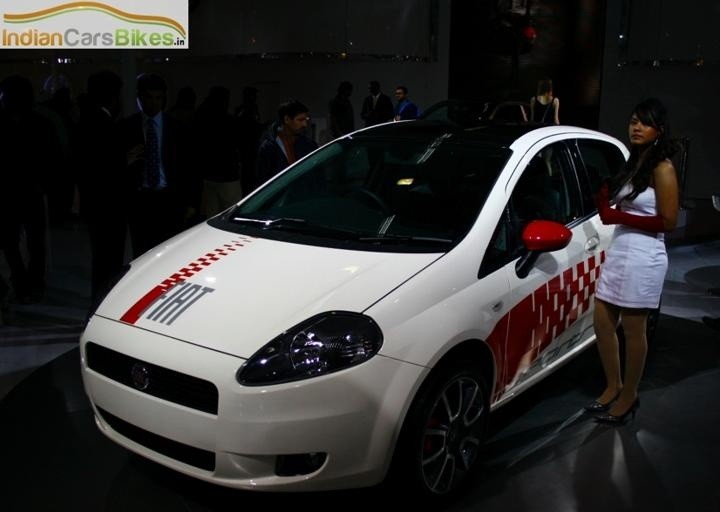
[597,184,664,233]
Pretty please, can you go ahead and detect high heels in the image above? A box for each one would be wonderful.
[593,395,640,424]
[585,391,620,411]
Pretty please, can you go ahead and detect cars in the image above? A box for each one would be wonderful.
[80,106,646,500]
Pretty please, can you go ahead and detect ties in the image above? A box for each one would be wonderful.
[146,120,159,187]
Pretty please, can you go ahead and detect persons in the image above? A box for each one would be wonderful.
[328,80,355,138]
[360,79,394,127]
[1,69,320,306]
[580,97,680,425]
[392,84,418,122]
[531,73,563,127]
[323,140,385,230]
[491,197,544,254]
